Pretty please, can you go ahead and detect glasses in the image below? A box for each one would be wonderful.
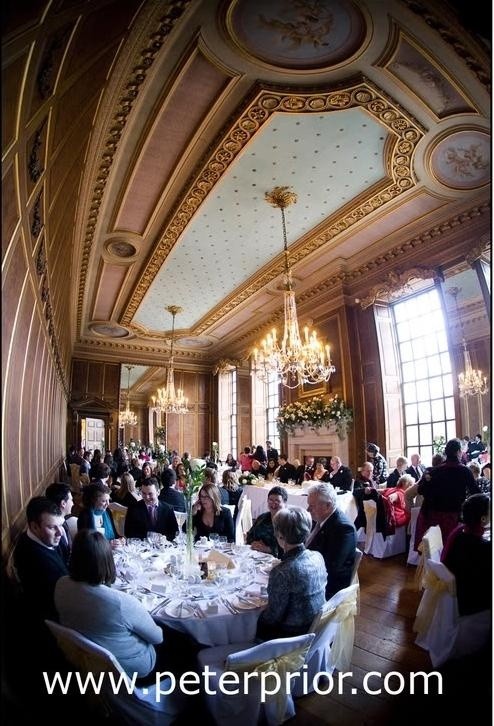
[200,495,210,499]
[268,496,286,505]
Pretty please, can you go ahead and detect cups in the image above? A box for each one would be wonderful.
[143,532,158,552]
[230,542,241,556]
[209,533,219,549]
[126,537,140,551]
[217,536,227,550]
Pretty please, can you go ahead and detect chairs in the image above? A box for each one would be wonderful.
[44,619,181,726]
[198,633,315,725]
[363,500,405,558]
[235,495,251,544]
[408,507,422,565]
[311,584,358,668]
[107,501,128,538]
[414,560,491,668]
[419,523,443,591]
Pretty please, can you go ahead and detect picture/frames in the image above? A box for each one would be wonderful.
[298,375,328,399]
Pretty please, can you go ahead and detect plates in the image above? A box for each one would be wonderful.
[164,604,195,619]
[231,597,268,610]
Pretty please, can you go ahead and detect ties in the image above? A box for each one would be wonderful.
[305,521,320,548]
[148,505,159,532]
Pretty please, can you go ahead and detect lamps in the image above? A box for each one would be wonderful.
[446,286,490,397]
[151,306,190,416]
[253,186,339,389]
[120,365,137,429]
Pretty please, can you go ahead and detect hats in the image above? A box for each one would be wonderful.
[367,443,380,456]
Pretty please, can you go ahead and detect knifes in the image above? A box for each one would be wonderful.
[177,602,184,619]
[234,594,259,608]
[220,597,241,616]
[149,598,171,616]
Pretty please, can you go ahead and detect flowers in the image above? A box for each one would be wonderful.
[277,394,354,437]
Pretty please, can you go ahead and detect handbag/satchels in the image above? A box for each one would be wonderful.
[384,488,411,526]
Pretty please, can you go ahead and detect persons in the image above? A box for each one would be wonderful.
[266,441,278,467]
[69,445,181,474]
[156,468,186,513]
[125,479,179,540]
[238,447,253,473]
[202,452,210,462]
[55,529,183,689]
[42,482,75,546]
[206,463,218,471]
[180,482,236,544]
[255,507,328,640]
[325,456,353,491]
[91,463,112,484]
[405,455,426,480]
[310,457,329,482]
[475,434,485,451]
[353,462,375,527]
[460,436,469,456]
[181,452,193,476]
[386,457,408,488]
[412,437,481,556]
[176,464,189,490]
[111,472,138,509]
[250,460,265,480]
[199,468,230,505]
[477,450,490,480]
[439,493,492,618]
[364,443,389,484]
[404,455,445,535]
[226,454,236,466]
[293,456,317,486]
[273,458,300,481]
[465,461,491,498]
[242,446,266,463]
[276,455,296,483]
[244,487,286,558]
[79,481,119,541]
[303,483,359,599]
[220,461,241,505]
[266,459,278,480]
[137,462,159,486]
[217,470,245,526]
[469,443,480,459]
[15,495,72,621]
[460,452,472,464]
[365,474,414,528]
